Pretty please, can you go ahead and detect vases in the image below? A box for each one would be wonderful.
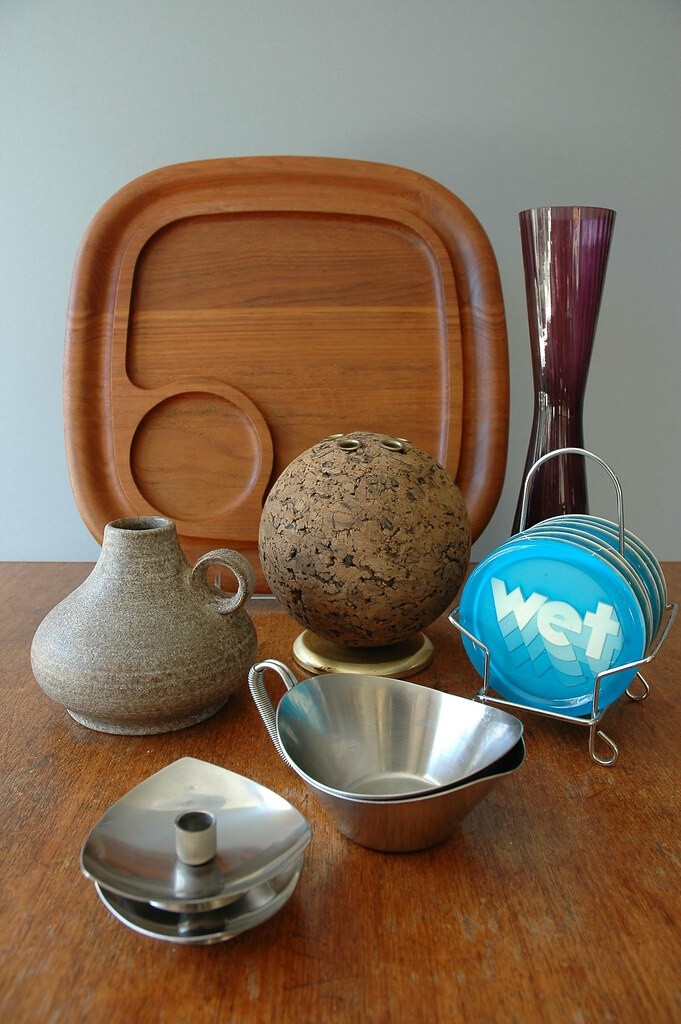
[511,204,617,538]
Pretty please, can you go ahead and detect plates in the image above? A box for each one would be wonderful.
[459,515,667,717]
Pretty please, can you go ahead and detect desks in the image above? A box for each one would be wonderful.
[0,562,681,1024]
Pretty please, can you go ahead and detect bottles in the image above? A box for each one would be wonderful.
[30,516,257,736]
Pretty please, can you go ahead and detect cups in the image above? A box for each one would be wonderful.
[248,658,526,853]
